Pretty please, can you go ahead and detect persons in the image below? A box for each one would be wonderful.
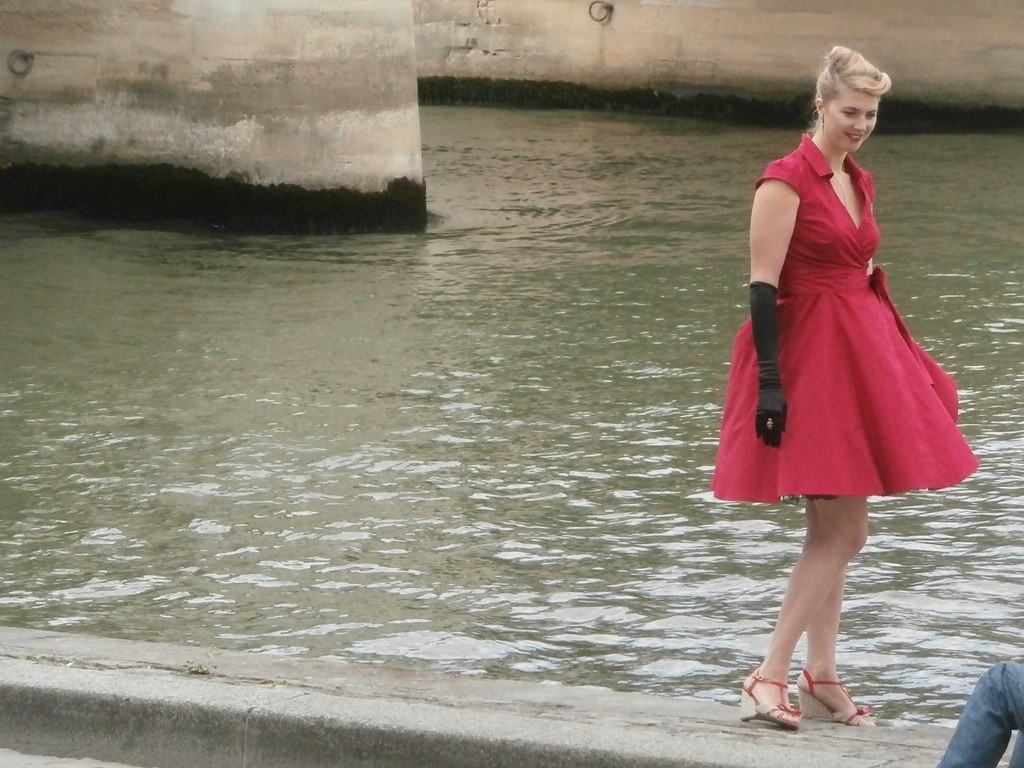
[937,661,1024,768]
[709,44,980,727]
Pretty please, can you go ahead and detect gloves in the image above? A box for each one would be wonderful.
[746,280,787,447]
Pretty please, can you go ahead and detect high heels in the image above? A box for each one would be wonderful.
[738,669,803,731]
[795,671,878,730]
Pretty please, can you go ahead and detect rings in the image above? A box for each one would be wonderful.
[766,418,774,430]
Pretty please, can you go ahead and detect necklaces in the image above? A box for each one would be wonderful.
[830,165,848,207]
